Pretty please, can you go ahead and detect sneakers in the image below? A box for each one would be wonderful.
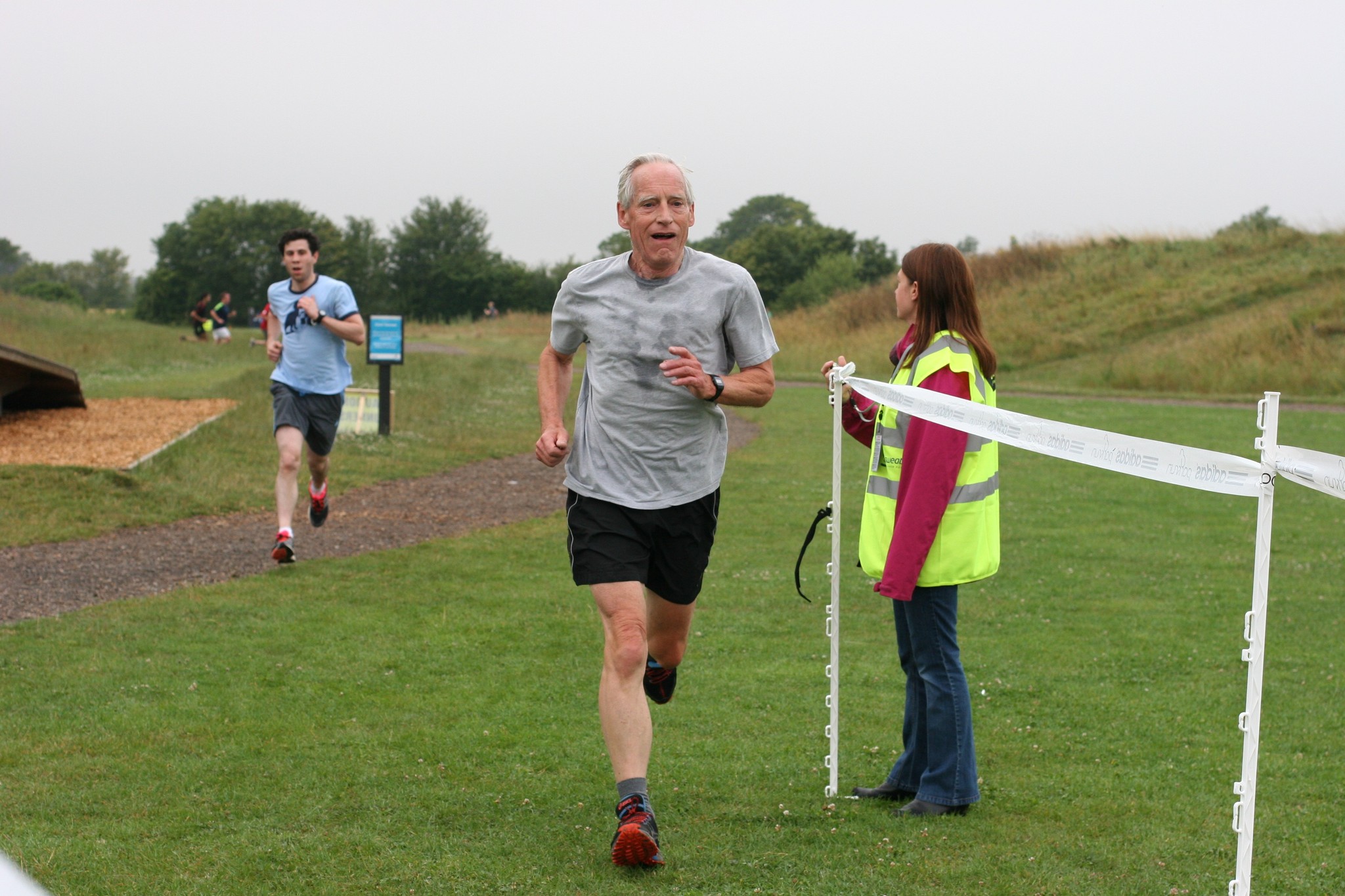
[610,794,665,867]
[270,530,295,564]
[309,477,329,527]
[644,653,677,705]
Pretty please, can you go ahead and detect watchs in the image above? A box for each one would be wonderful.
[704,374,725,402]
[314,309,326,324]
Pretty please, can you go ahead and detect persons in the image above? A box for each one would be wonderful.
[483,301,499,321]
[533,158,780,866]
[248,229,366,564]
[179,291,211,343]
[819,243,1002,815]
[210,292,237,347]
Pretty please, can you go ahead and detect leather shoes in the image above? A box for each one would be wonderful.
[852,781,916,799]
[891,799,968,817]
